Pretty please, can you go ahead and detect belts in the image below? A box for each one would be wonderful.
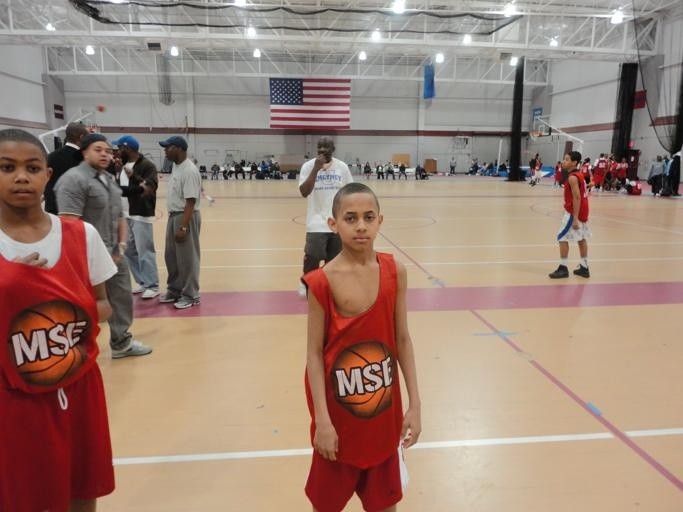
[168,210,199,217]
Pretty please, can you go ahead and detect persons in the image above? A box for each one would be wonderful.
[301,183,422,512]
[1,130,118,510]
[299,136,355,302]
[158,136,202,308]
[107,144,123,177]
[53,134,153,358]
[211,155,280,180]
[44,123,94,216]
[113,137,161,298]
[528,152,674,197]
[549,151,589,278]
[363,161,429,180]
[192,159,215,208]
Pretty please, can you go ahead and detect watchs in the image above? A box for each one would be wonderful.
[179,226,187,231]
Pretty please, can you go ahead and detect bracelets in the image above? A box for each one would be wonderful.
[119,242,128,251]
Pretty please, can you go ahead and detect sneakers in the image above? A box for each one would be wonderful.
[160,292,181,303]
[141,286,160,298]
[132,284,145,294]
[111,339,153,359]
[548,264,569,279]
[573,264,590,278]
[173,295,201,309]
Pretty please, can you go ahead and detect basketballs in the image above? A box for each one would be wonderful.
[330,342,394,418]
[96,104,105,112]
[8,300,91,388]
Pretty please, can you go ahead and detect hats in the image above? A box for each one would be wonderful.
[111,135,139,152]
[159,136,188,151]
[80,133,107,151]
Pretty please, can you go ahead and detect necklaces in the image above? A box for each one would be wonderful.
[320,159,333,171]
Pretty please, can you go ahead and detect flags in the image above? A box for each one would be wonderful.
[270,79,351,130]
